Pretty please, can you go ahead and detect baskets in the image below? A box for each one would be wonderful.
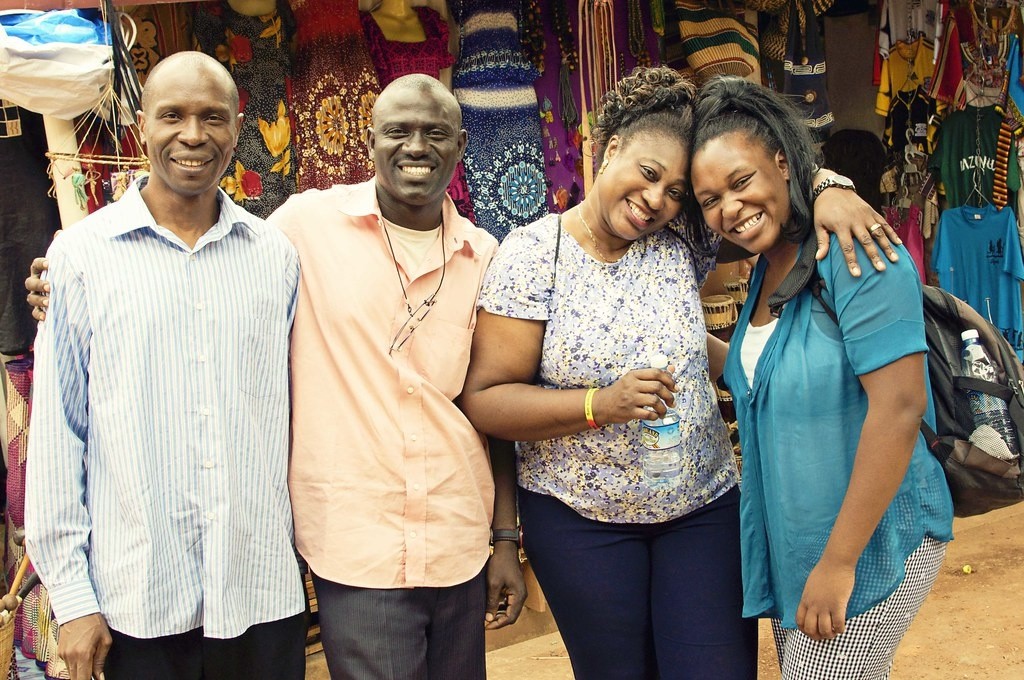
[0,618,15,680]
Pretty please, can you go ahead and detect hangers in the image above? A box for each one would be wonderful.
[899,24,999,210]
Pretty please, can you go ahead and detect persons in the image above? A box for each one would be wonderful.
[26,73,528,680]
[690,79,953,680]
[458,66,902,680]
[28,51,301,680]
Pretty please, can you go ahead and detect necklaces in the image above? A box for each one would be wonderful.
[578,201,621,262]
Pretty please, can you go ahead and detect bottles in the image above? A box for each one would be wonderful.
[640,354,682,491]
[960,329,1022,460]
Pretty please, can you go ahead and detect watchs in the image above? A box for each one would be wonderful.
[813,175,857,201]
[490,524,524,549]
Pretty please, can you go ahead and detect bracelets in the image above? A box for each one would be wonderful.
[585,388,604,431]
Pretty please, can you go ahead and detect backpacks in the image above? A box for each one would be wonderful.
[768,227,1024,518]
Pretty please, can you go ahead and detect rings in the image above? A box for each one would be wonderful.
[869,223,883,233]
